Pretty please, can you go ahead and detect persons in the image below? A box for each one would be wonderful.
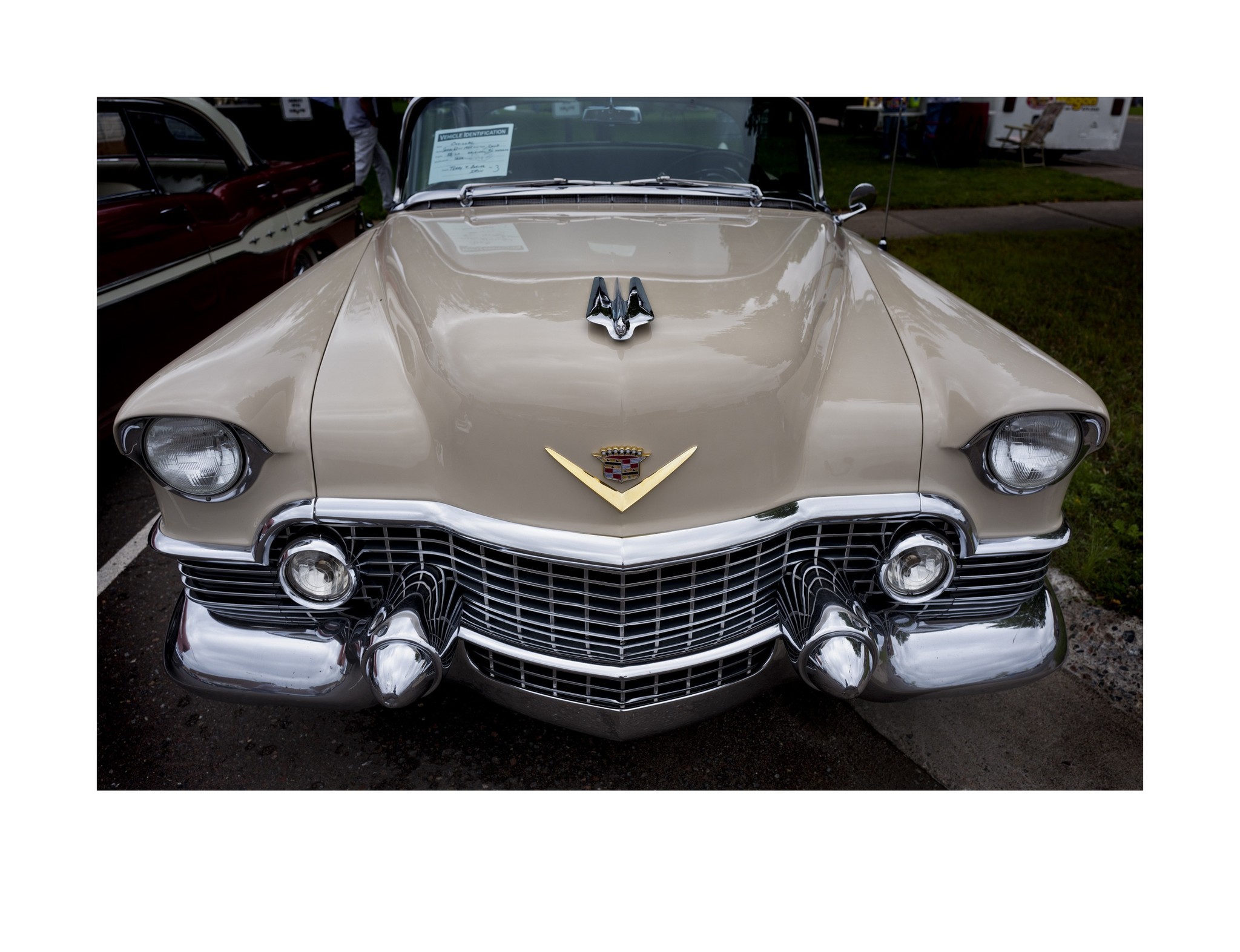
[339,97,393,210]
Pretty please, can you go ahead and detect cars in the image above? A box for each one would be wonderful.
[97,97,374,430]
[114,97,1109,741]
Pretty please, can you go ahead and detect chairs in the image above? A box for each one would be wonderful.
[996,101,1065,168]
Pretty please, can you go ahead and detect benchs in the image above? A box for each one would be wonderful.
[511,142,704,181]
